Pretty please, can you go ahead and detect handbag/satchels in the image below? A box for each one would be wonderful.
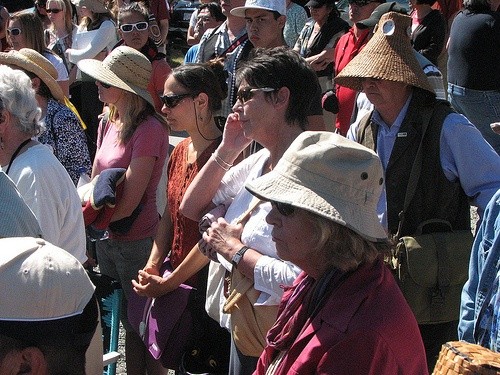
[429,340,500,375]
[127,253,200,370]
[176,285,231,375]
[222,199,282,358]
[391,218,474,324]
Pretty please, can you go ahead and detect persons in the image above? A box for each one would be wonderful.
[0,0,500,375]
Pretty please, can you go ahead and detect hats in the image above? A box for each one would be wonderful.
[356,1,408,31]
[230,0,286,18]
[35,0,47,8]
[0,47,65,102]
[64,20,118,65]
[304,0,335,9]
[70,0,110,13]
[77,45,156,113]
[244,130,390,243]
[332,11,438,97]
[0,236,97,322]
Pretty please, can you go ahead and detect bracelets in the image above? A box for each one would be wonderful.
[212,153,229,171]
[214,149,233,167]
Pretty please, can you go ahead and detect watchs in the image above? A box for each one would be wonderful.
[231,245,250,269]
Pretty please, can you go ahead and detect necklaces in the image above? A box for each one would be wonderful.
[114,118,124,132]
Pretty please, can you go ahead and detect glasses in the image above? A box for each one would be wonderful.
[270,198,296,216]
[358,75,383,81]
[307,4,326,9]
[95,80,113,89]
[348,0,380,7]
[6,28,22,37]
[46,8,63,14]
[119,21,149,33]
[159,89,202,109]
[235,86,279,104]
